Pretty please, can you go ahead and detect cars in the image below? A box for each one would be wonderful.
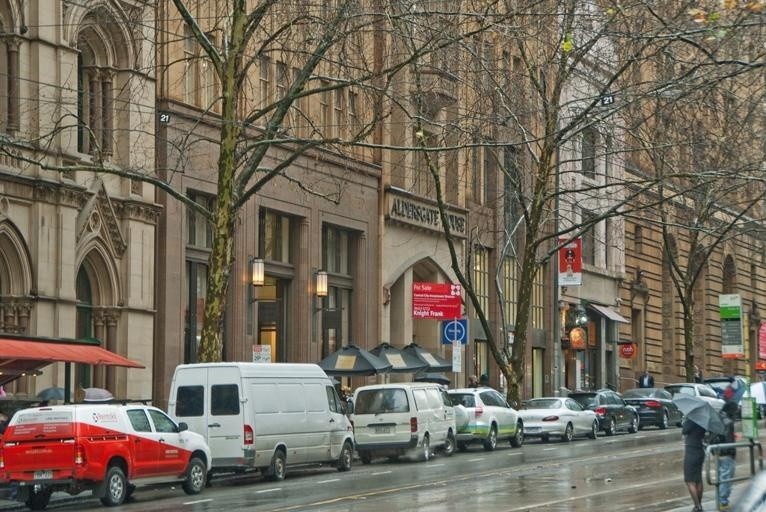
[625,375,766,430]
[518,395,600,442]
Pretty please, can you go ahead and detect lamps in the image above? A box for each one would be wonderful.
[313,268,329,297]
[250,255,265,287]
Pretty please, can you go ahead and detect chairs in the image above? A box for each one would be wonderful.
[383,398,397,412]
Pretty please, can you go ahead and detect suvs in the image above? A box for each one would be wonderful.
[567,388,640,436]
[2,405,215,509]
[447,385,526,453]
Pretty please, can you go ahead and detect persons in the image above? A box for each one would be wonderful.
[693,363,702,381]
[680,417,706,511]
[707,401,738,511]
[466,375,481,387]
[639,367,654,389]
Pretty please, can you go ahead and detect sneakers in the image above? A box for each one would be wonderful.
[720,502,732,511]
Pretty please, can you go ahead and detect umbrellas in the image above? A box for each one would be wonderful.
[81,387,115,400]
[732,381,765,405]
[369,340,431,384]
[36,383,73,400]
[317,342,396,383]
[671,391,729,438]
[404,343,452,383]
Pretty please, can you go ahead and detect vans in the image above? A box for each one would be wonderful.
[166,359,356,481]
[350,381,456,459]
